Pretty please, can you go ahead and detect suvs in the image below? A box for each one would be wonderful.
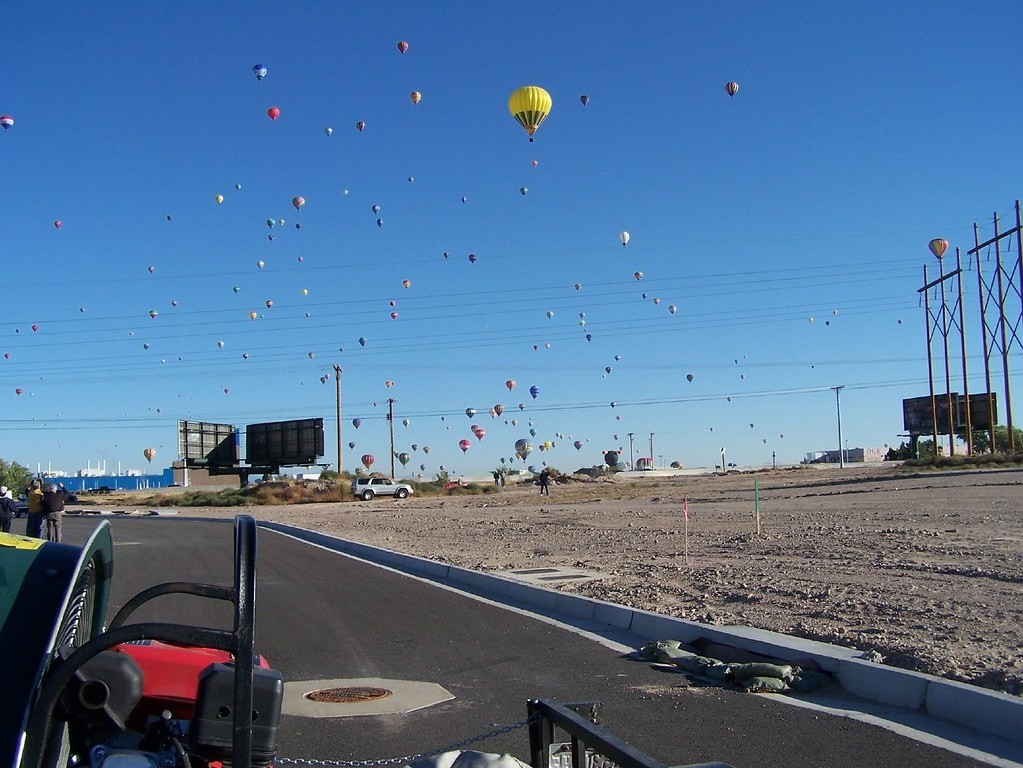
[351,476,414,501]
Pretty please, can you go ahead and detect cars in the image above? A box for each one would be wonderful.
[14,493,29,518]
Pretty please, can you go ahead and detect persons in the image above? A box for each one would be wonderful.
[0,481,72,543]
[540,469,551,496]
[459,471,507,488]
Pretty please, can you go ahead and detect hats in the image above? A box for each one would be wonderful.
[0,486,8,493]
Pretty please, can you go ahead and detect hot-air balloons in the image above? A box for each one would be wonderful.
[507,85,553,143]
[253,64,268,83]
[578,95,592,110]
[0,157,904,478]
[928,237,949,263]
[267,107,280,121]
[356,121,365,133]
[144,448,156,464]
[399,453,410,467]
[353,419,361,430]
[0,114,15,132]
[398,41,409,55]
[515,439,533,463]
[54,220,62,229]
[292,196,306,211]
[459,439,471,453]
[724,81,739,98]
[325,126,335,137]
[410,91,422,106]
[361,455,375,469]
